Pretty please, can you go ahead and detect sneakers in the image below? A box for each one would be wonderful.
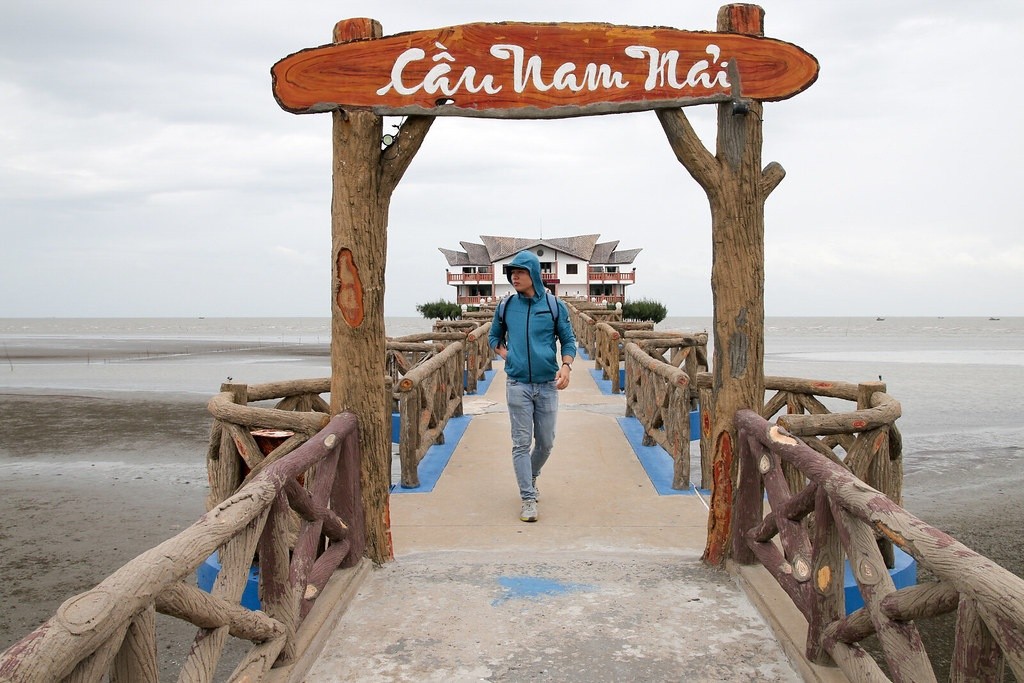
[520,484,542,522]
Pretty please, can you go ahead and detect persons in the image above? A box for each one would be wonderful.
[488,250,576,523]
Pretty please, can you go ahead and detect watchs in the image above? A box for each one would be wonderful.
[561,362,572,371]
